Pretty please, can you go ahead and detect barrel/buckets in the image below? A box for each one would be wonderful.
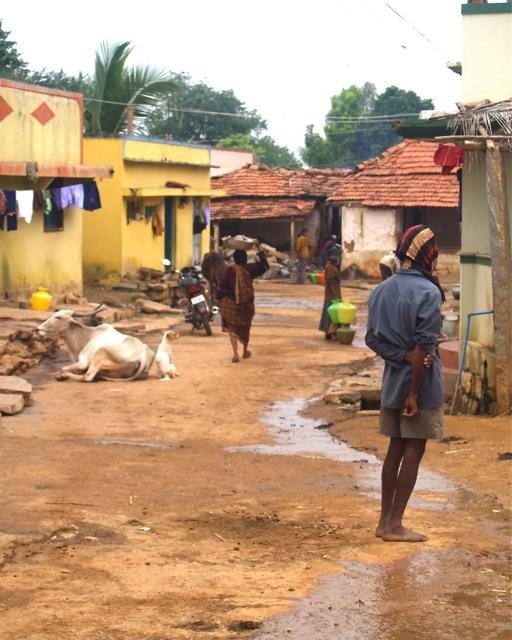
[32,287,52,312]
[327,300,358,344]
[310,269,324,283]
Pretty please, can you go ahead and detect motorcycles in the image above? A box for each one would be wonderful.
[162,256,220,336]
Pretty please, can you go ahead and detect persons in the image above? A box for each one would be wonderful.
[379,250,400,283]
[364,224,444,543]
[317,234,341,271]
[293,227,312,285]
[211,241,270,364]
[318,250,342,342]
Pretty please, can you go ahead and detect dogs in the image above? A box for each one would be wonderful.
[155,328,180,381]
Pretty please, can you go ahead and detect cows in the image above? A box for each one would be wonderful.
[202,253,230,305]
[36,303,155,382]
